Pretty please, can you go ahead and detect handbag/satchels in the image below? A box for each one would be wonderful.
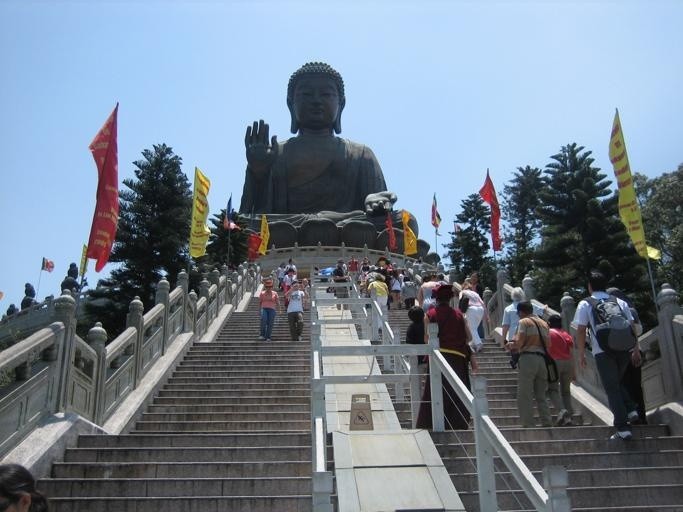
[458,295,469,313]
[543,352,558,383]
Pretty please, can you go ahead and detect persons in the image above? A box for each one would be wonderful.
[416,284,473,430]
[238,62,418,238]
[548,315,577,425]
[570,272,643,441]
[0,463,49,512]
[606,287,647,425]
[61,263,88,293]
[258,255,549,373]
[21,283,40,310]
[506,301,553,427]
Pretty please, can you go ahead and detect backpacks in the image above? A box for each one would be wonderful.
[361,265,369,273]
[584,295,637,354]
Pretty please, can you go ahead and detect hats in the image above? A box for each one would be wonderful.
[431,284,455,301]
[337,257,345,264]
[368,265,385,282]
[262,279,273,286]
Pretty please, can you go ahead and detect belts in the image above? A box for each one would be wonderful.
[519,351,543,356]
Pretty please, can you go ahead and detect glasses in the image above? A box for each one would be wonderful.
[265,286,272,288]
[0,490,18,511]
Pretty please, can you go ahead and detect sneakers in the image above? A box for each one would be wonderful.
[562,416,572,426]
[257,335,265,340]
[610,429,633,441]
[626,408,639,422]
[265,337,271,341]
[296,334,302,341]
[555,408,568,425]
[468,341,482,353]
